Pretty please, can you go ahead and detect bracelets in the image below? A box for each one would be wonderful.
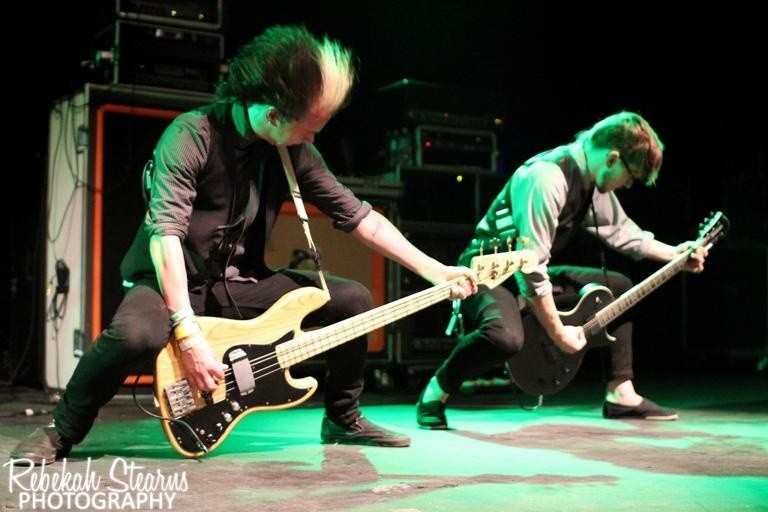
[170,305,203,351]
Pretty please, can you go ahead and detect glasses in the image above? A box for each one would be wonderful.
[605,150,641,182]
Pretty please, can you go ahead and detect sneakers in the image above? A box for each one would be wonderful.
[602,394,679,421]
[8,424,73,467]
[415,386,449,430]
[319,413,411,448]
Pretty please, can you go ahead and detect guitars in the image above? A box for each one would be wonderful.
[151,235,539,458]
[504,210,729,394]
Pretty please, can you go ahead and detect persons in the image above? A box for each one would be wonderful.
[415,109,708,431]
[9,26,478,466]
